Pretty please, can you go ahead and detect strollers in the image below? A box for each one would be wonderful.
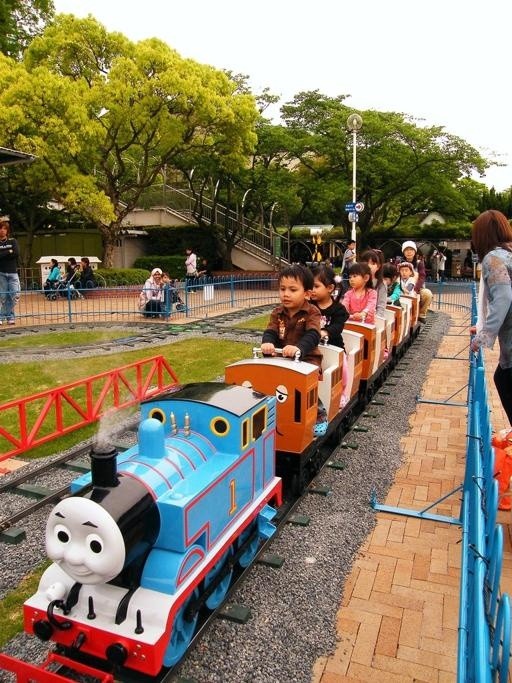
[170,279,187,311]
[46,265,80,300]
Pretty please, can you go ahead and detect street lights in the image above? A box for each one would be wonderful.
[348,112,363,263]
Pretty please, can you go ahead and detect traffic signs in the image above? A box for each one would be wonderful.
[346,203,364,213]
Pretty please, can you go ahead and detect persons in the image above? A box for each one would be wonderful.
[184,259,197,289]
[463,253,472,281]
[198,260,211,290]
[470,210,512,429]
[186,248,197,292]
[262,240,447,423]
[139,268,165,318]
[43,258,96,300]
[0,221,21,324]
[161,272,181,305]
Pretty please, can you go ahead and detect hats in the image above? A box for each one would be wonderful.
[401,240,417,252]
[397,262,415,277]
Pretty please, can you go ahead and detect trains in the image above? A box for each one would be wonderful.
[0,289,421,681]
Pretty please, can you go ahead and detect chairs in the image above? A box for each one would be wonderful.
[316,293,420,424]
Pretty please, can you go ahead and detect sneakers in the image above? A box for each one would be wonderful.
[7,319,15,325]
